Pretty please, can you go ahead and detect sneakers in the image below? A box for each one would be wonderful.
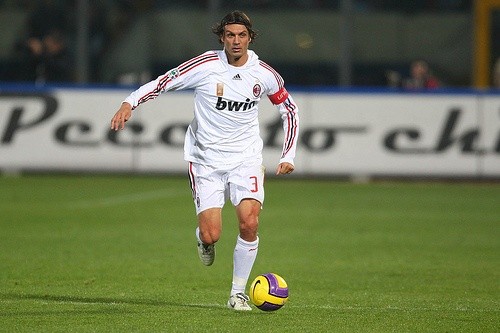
[196,226,216,267]
[227,292,252,311]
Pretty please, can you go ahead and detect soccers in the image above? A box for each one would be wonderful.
[248,272,289,312]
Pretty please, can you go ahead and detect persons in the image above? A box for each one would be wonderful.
[24,37,43,85]
[404,59,441,91]
[40,36,63,83]
[110,10,300,311]
